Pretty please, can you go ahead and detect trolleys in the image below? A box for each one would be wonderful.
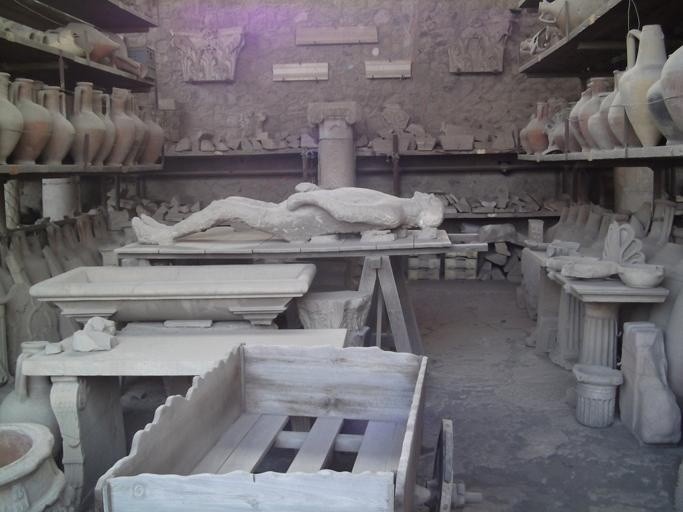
[94,342,482,512]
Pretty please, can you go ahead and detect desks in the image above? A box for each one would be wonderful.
[113,226,450,357]
[520,246,670,376]
[21,327,347,507]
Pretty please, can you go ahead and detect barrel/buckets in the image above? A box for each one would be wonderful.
[41,177,77,223]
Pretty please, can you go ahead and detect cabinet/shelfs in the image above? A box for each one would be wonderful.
[509,1,682,162]
[0,0,168,176]
[353,148,569,220]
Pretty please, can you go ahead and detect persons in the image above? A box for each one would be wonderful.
[130,182,446,243]
